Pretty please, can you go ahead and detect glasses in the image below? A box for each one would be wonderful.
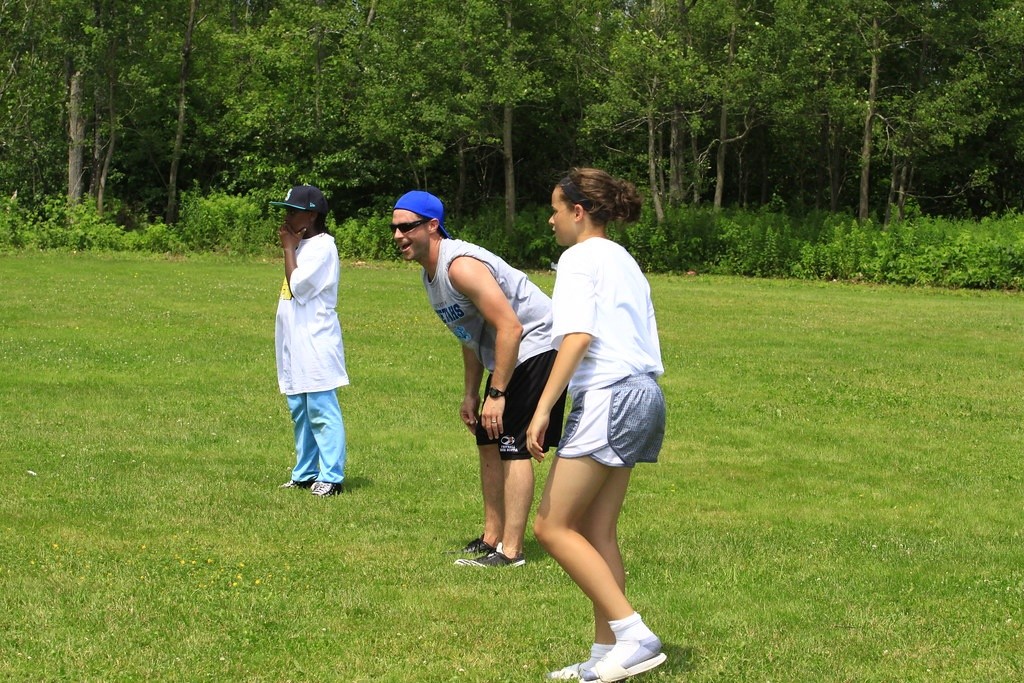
[388,218,431,232]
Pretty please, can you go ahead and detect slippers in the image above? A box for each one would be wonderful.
[545,659,585,680]
[578,652,667,682]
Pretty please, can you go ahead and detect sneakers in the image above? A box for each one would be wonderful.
[311,482,342,498]
[453,542,525,569]
[443,531,496,555]
[277,477,315,490]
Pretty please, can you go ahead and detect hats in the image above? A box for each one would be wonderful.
[393,189,449,238]
[267,184,328,216]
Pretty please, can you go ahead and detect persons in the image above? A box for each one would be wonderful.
[268,185,350,497]
[392,192,568,569]
[525,169,666,683]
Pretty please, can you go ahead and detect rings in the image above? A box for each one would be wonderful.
[492,421,497,423]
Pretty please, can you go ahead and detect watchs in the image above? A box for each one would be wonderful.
[489,387,505,398]
